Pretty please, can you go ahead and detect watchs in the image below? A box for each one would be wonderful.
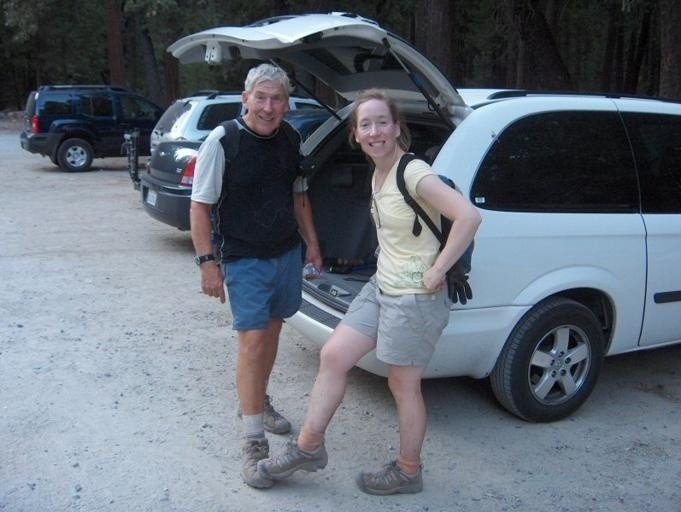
[194,254,216,266]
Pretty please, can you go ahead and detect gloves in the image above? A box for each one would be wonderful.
[446,268,472,304]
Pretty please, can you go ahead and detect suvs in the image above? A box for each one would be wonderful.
[168,12,681,423]
[21,84,166,172]
[118,87,334,192]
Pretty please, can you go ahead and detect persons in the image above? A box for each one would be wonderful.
[256,90,483,497]
[190,62,324,489]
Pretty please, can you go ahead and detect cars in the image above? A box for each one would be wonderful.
[139,95,340,230]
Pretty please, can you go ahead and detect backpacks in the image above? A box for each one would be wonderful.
[396,152,475,273]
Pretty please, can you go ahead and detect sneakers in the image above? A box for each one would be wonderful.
[357,459,424,495]
[238,393,291,434]
[243,435,274,488]
[257,435,329,480]
